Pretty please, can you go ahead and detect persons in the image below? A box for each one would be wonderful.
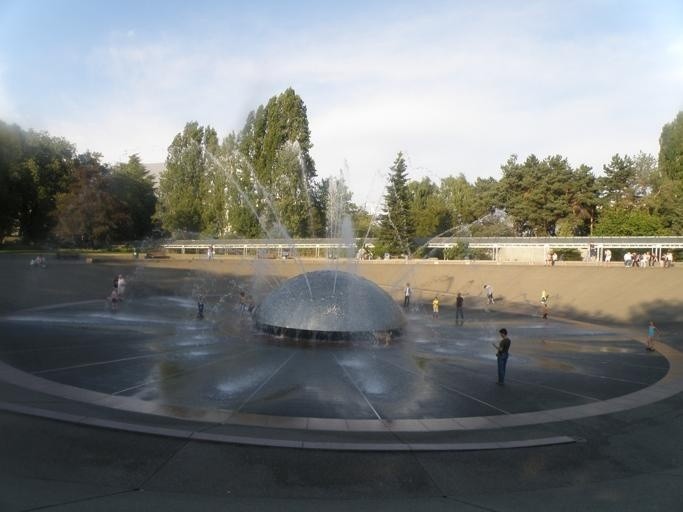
[358,246,373,261]
[197,289,257,321]
[484,284,495,304]
[106,271,126,313]
[432,297,439,319]
[544,251,564,266]
[493,328,511,384]
[208,246,215,261]
[540,289,547,304]
[405,283,413,307]
[605,250,611,263]
[457,293,463,320]
[624,251,673,268]
[646,320,661,351]
[543,304,550,318]
[30,255,46,266]
